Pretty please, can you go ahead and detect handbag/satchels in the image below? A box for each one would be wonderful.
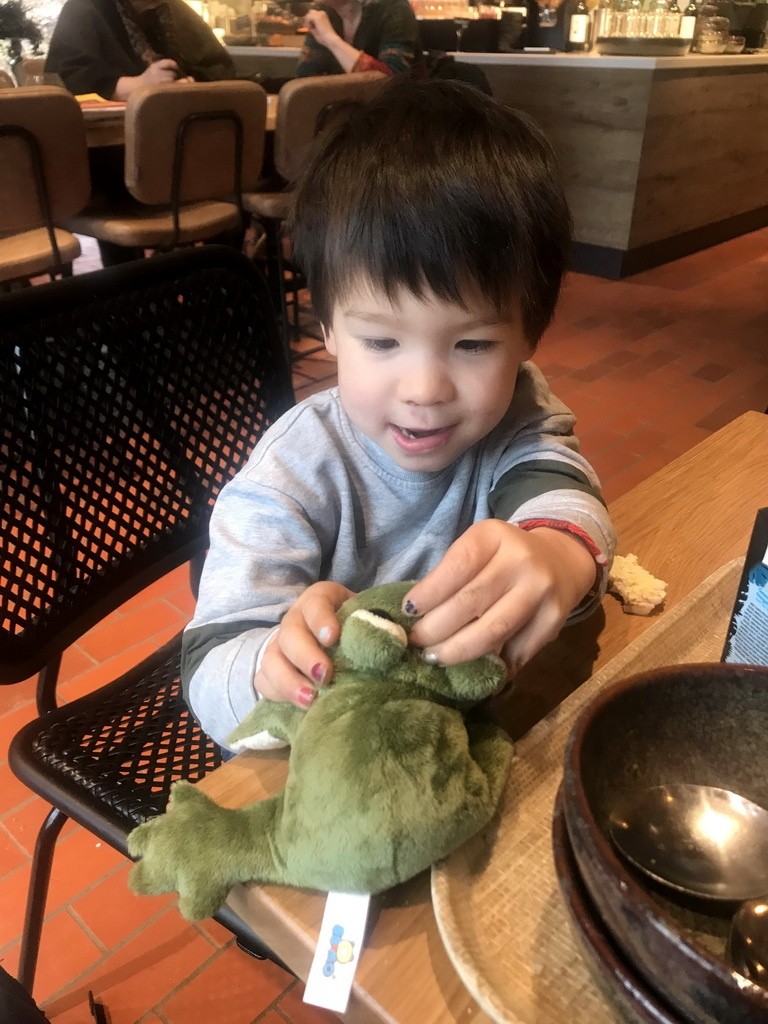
[412,47,492,97]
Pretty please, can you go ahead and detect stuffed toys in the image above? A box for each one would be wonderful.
[124,580,511,919]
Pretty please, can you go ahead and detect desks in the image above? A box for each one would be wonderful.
[168,407,768,1024]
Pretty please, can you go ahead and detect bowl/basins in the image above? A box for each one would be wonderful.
[549,661,768,1024]
[698,17,745,55]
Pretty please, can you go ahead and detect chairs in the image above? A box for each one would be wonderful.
[0,60,391,1024]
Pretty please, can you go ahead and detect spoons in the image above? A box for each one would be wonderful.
[607,784,767,902]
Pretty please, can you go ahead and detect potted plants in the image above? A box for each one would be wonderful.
[0,0,45,67]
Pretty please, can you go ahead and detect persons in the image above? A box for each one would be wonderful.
[181,77,618,762]
[513,0,591,53]
[295,0,417,80]
[43,1,239,269]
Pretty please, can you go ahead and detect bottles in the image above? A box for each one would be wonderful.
[557,0,696,52]
